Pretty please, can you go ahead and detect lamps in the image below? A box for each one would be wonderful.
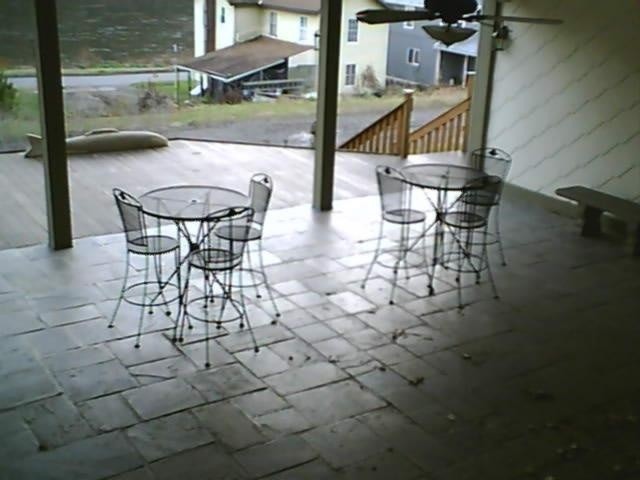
[490,24,514,51]
[422,24,477,46]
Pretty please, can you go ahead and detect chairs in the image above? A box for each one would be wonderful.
[106,184,193,349]
[207,173,282,319]
[427,176,502,307]
[360,165,435,303]
[451,147,513,283]
[173,204,260,368]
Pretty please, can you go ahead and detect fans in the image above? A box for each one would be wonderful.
[353,0,565,26]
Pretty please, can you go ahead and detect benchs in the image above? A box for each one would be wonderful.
[553,184,639,254]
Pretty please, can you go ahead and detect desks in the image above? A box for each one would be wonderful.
[139,186,250,308]
[400,164,487,268]
